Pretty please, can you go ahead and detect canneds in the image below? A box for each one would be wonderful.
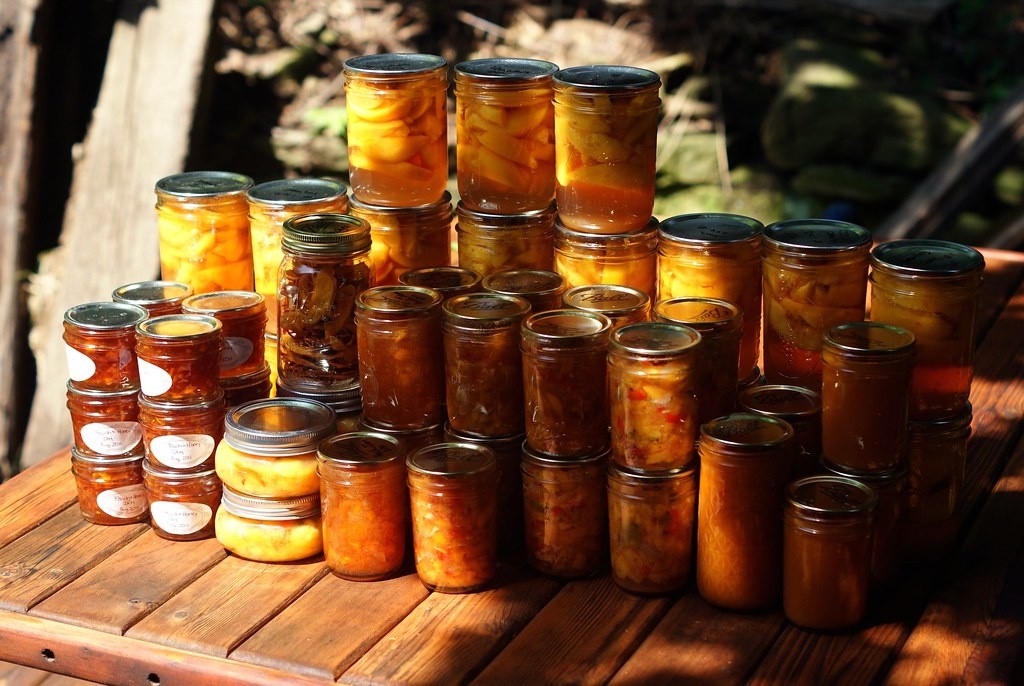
[63,54,984,629]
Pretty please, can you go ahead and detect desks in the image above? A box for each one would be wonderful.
[0,281,1024,686]
